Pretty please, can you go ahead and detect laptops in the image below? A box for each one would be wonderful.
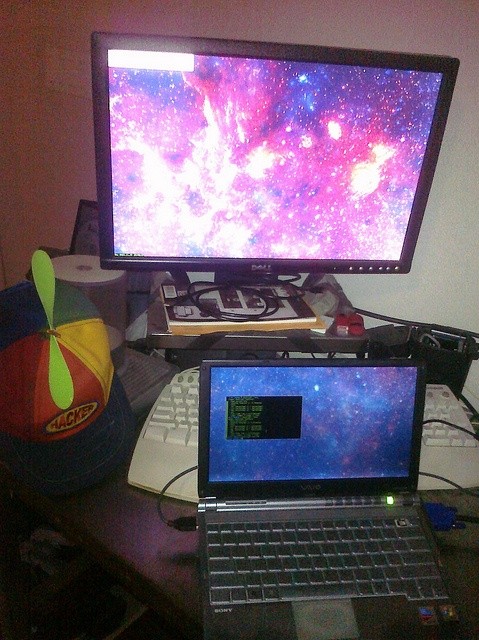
[197,358,468,640]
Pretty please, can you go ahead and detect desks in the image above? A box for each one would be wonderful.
[0,280,479,640]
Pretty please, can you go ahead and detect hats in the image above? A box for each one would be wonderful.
[0,278,137,500]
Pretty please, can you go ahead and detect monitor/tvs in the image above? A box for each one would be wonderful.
[91,30,460,286]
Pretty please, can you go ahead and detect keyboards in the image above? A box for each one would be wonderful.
[128,366,478,503]
